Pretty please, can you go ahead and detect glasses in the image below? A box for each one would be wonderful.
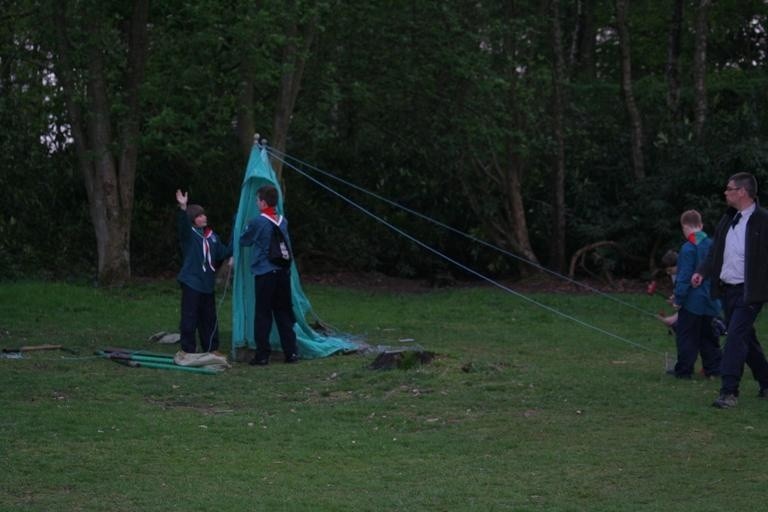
[726,187,741,191]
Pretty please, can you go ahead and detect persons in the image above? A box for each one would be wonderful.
[690,173,768,408]
[662,248,727,336]
[669,209,725,379]
[238,185,299,365]
[175,188,233,354]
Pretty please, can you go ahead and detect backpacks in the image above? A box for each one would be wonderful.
[256,223,292,267]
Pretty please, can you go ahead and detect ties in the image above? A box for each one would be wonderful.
[731,213,741,229]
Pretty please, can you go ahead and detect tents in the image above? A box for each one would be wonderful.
[229,139,354,357]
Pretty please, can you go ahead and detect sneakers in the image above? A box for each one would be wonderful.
[666,368,692,379]
[712,395,738,408]
[757,388,768,399]
[251,355,299,365]
